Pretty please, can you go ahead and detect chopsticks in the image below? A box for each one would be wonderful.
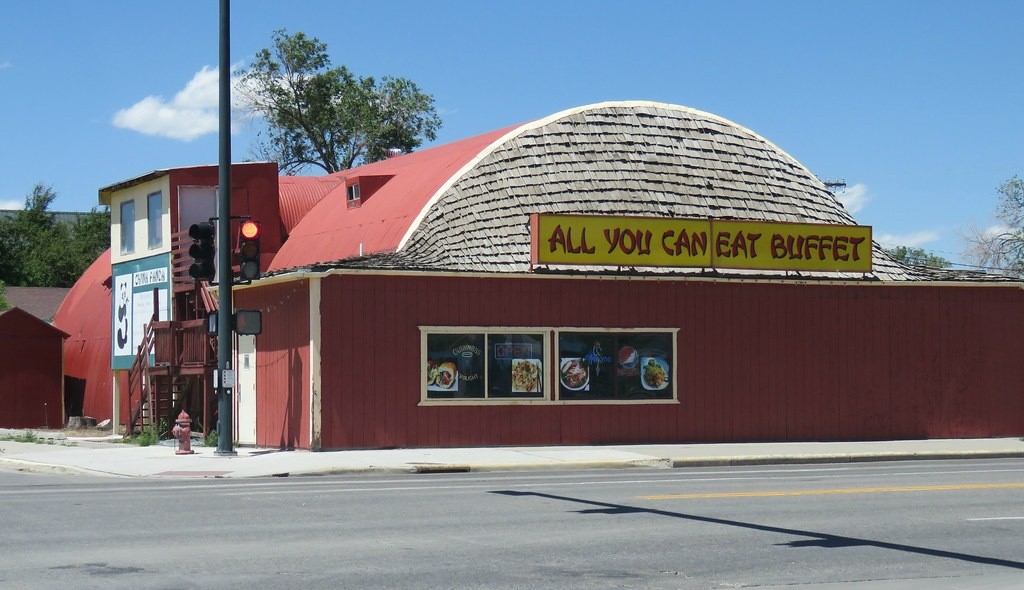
[536,361,542,392]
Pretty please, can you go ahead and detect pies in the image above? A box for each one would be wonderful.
[427,361,457,388]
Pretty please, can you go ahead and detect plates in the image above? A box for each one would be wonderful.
[641,365,669,390]
[512,359,542,392]
[427,370,458,391]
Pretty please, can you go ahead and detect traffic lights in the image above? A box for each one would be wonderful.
[236,310,263,335]
[187,220,216,281]
[239,219,260,280]
[206,310,218,336]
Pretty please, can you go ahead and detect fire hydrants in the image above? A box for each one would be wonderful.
[172,411,195,454]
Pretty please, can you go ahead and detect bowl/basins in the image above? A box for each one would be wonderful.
[561,358,589,390]
